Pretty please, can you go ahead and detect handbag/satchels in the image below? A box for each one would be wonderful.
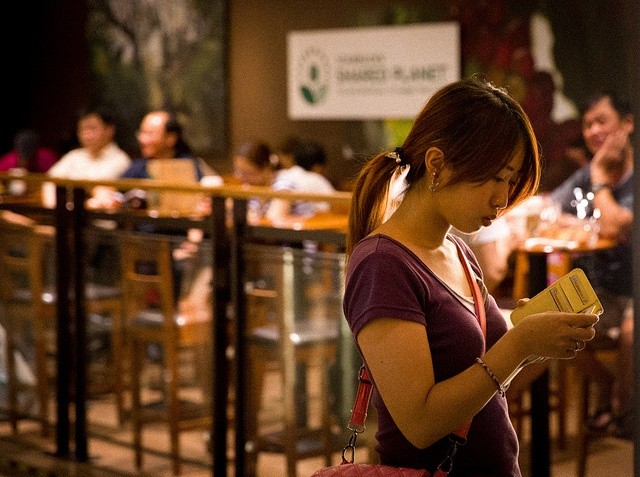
[312,464,452,477]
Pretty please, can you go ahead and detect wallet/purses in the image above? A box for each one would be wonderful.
[509,268,604,364]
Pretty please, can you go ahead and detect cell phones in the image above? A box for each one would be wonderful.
[519,306,606,366]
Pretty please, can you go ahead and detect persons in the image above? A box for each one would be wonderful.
[118,109,224,274]
[232,137,277,185]
[308,141,337,190]
[0,134,59,174]
[46,105,133,181]
[343,79,599,476]
[264,135,336,219]
[548,89,636,437]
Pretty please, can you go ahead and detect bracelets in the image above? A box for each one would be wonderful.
[590,181,611,194]
[475,356,503,394]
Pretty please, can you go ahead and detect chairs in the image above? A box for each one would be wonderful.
[242,243,336,477]
[0,233,126,438]
[507,253,568,451]
[115,228,216,477]
